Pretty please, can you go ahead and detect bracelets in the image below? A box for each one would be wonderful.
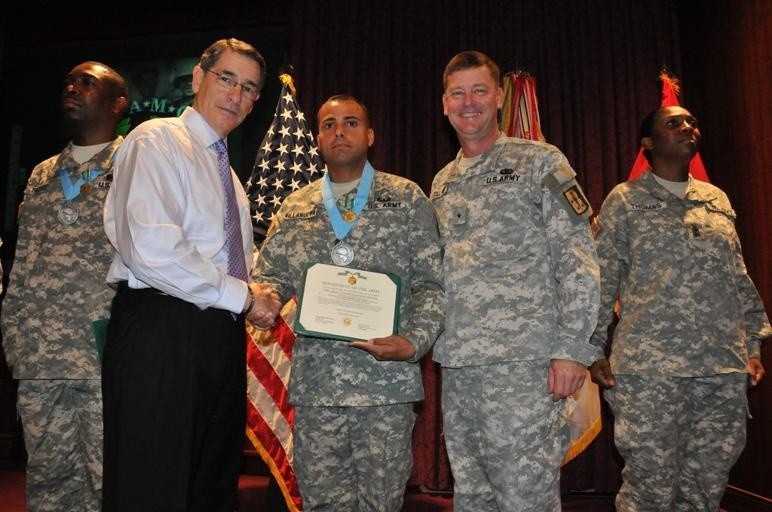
[245,285,255,314]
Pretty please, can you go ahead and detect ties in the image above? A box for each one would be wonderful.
[210,138,248,322]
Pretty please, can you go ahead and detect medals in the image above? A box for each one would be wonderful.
[58,203,79,225]
[331,242,354,266]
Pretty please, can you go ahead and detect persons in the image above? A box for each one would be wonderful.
[102,36,281,512]
[1,62,128,511]
[248,93,444,511]
[589,105,772,511]
[429,51,601,512]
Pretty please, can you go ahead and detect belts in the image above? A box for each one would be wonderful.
[114,280,169,296]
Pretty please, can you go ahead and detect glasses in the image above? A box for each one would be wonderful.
[202,67,257,99]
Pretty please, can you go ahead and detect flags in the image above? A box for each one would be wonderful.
[498,72,600,469]
[615,74,709,317]
[245,87,326,511]
